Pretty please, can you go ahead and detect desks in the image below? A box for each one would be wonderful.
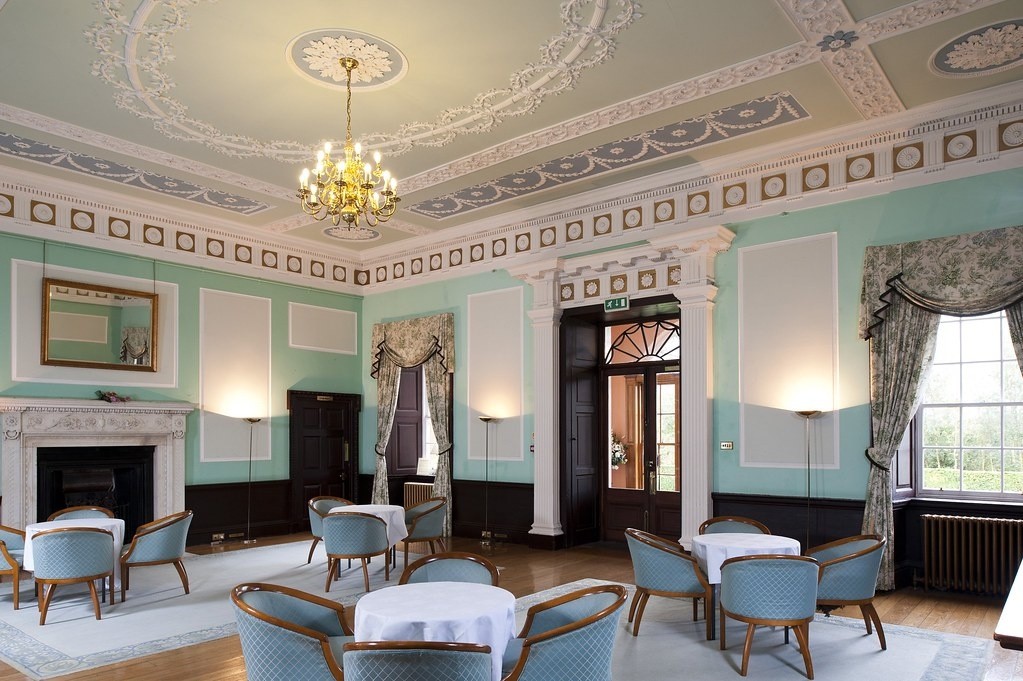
[23,518,125,604]
[328,505,408,578]
[354,581,517,681]
[691,533,801,645]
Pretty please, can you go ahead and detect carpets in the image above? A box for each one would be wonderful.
[517,578,997,681]
[0,540,506,680]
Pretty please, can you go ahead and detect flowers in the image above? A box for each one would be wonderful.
[611,429,628,470]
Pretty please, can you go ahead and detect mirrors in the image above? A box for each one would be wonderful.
[39,277,159,373]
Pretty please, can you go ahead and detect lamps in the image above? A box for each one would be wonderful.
[242,418,261,543]
[297,56,401,233]
[479,417,496,545]
[795,411,821,550]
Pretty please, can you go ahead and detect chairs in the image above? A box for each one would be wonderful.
[230,552,628,681]
[0,506,193,625]
[625,515,887,680]
[308,495,447,593]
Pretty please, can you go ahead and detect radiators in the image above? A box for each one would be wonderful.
[919,513,1023,595]
[403,482,434,509]
